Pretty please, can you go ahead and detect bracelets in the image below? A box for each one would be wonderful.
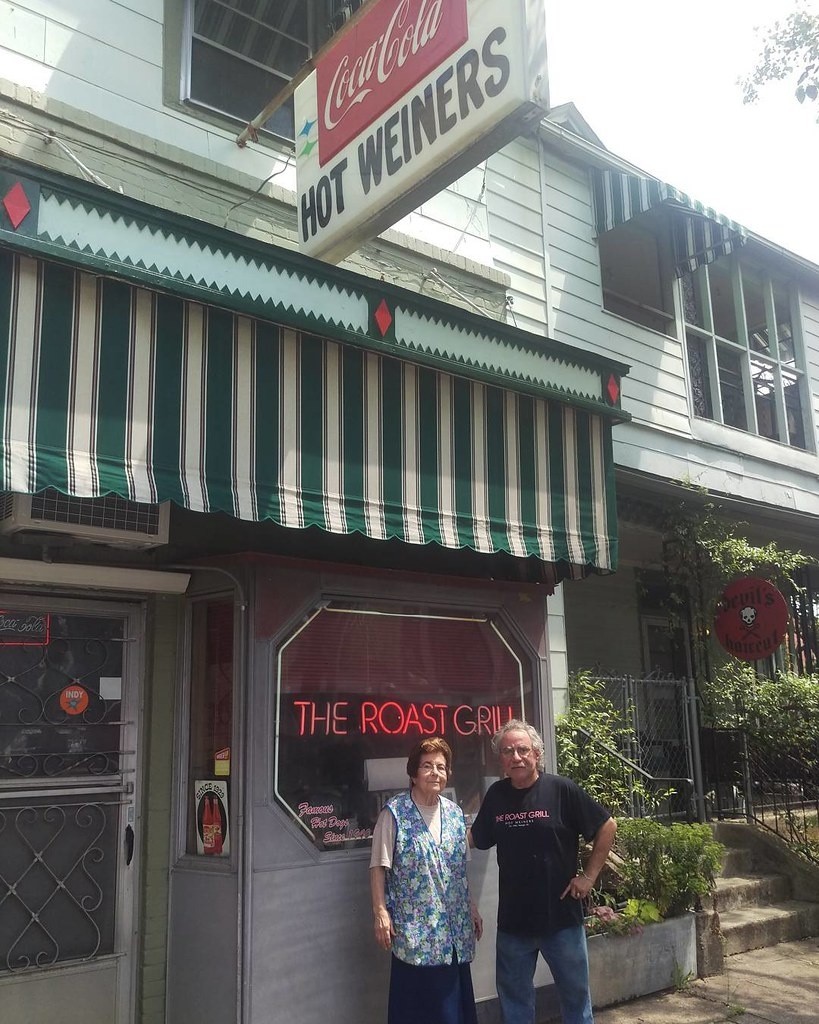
[581,872,592,881]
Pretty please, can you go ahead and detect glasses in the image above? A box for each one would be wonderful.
[500,746,533,758]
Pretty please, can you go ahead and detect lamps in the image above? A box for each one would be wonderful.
[664,540,685,587]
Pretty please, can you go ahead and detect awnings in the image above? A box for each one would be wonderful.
[0,241,623,574]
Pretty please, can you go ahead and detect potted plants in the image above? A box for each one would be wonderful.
[629,480,818,782]
[586,817,727,1012]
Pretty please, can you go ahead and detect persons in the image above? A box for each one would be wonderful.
[465,720,618,1024]
[368,736,484,1024]
